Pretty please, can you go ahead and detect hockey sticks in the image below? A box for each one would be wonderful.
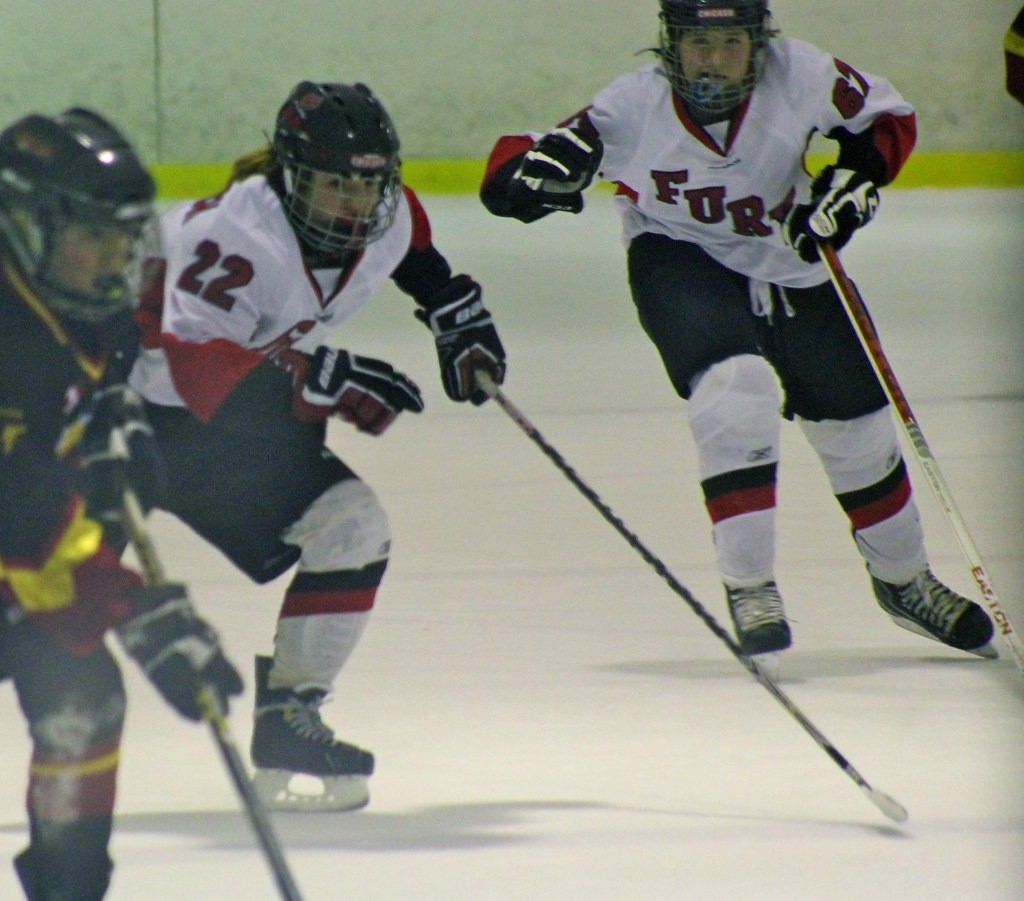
[818,237,1024,665]
[120,485,301,900]
[470,362,916,828]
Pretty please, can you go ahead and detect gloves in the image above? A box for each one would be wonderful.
[117,583,243,721]
[77,377,164,523]
[508,127,604,213]
[413,274,506,407]
[781,164,880,263]
[302,344,424,436]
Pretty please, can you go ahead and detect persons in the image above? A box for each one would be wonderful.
[0,82,508,901]
[1002,0,1024,107]
[479,0,995,655]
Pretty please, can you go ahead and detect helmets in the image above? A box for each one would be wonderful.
[656,0,768,115]
[0,107,157,334]
[273,81,399,267]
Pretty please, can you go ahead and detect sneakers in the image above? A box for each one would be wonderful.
[719,572,800,679]
[250,656,374,812]
[865,563,1000,660]
